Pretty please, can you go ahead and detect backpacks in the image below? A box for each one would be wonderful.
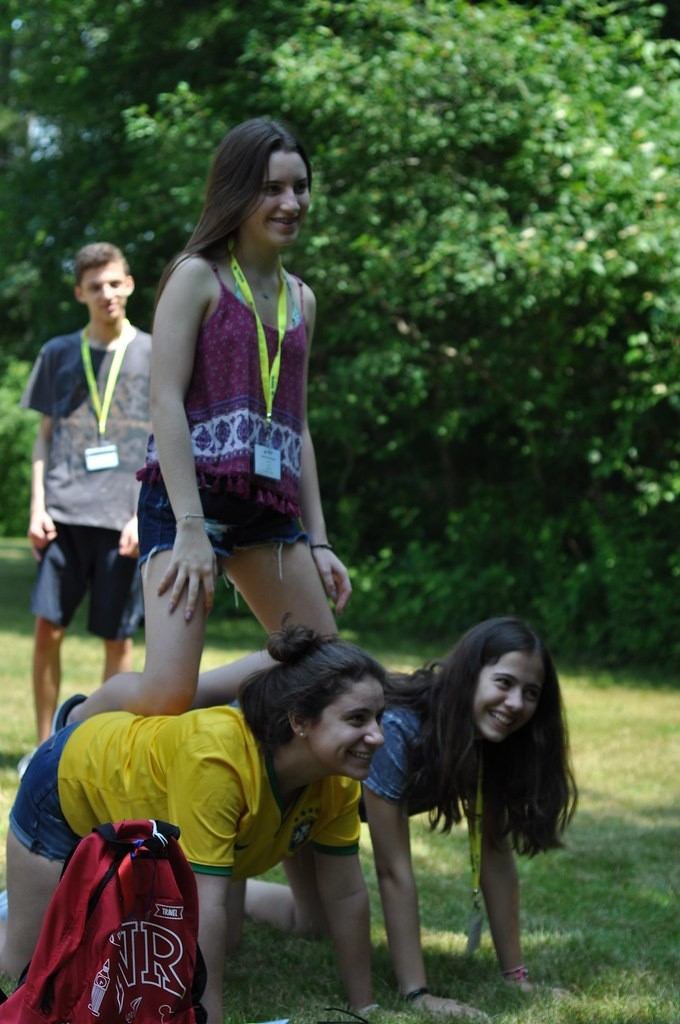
[1,820,213,1024]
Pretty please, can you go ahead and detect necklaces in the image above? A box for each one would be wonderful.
[260,289,273,299]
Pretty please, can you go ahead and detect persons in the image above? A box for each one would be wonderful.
[241,615,579,1020]
[0,620,389,1024]
[17,241,152,780]
[49,116,350,731]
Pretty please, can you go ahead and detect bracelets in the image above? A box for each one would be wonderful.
[505,967,529,980]
[402,987,428,1002]
[312,544,333,549]
[176,513,204,521]
[360,1003,381,1014]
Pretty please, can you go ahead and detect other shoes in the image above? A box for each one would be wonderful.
[51,694,87,736]
[17,750,36,779]
